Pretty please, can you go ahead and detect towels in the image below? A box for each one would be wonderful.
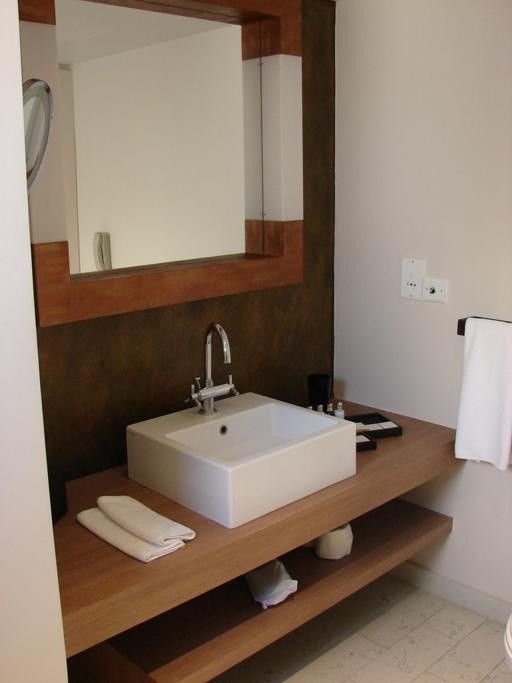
[453,317,511,471]
[75,492,197,566]
[240,558,301,608]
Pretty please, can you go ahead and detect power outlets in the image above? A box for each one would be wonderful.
[399,256,446,301]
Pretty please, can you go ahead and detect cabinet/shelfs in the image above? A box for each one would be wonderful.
[49,399,465,682]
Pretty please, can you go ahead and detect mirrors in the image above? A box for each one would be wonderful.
[19,0,303,332]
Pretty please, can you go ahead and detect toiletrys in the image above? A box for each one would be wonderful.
[308,401,345,420]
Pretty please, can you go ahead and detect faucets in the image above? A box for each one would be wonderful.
[184,320,240,416]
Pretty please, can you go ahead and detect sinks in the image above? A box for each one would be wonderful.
[124,390,356,530]
